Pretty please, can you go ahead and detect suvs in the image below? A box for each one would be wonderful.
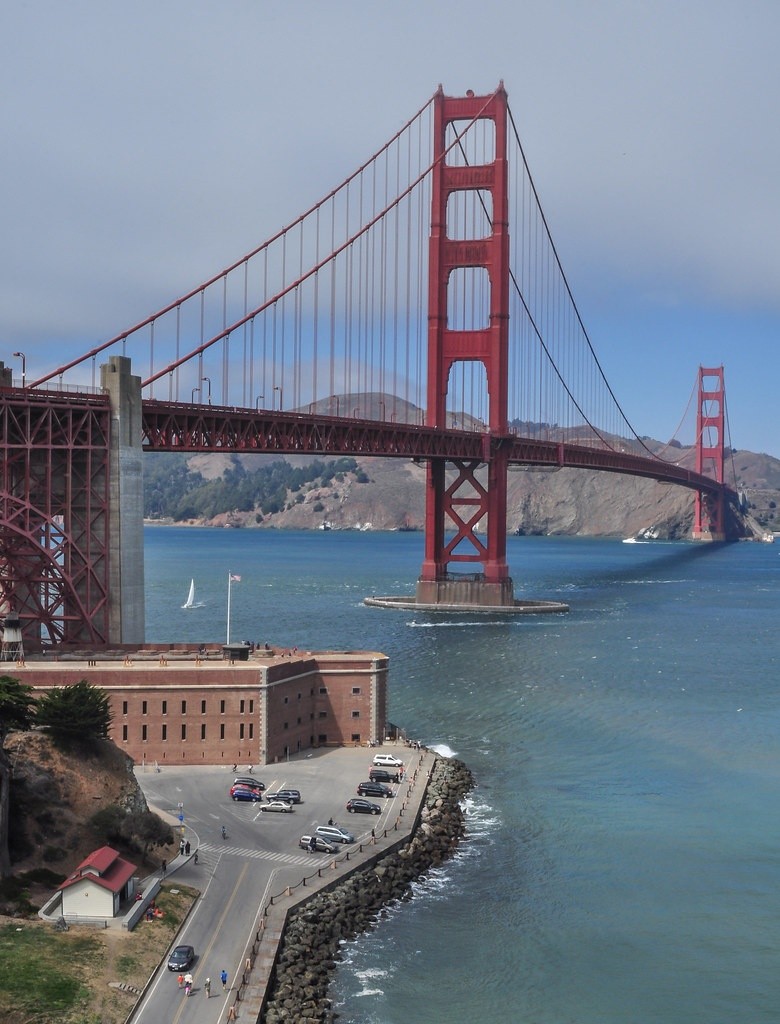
[267,790,301,805]
[373,754,403,767]
[346,798,381,816]
[369,769,397,784]
[356,782,392,799]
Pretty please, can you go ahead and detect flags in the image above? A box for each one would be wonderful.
[231,574,241,582]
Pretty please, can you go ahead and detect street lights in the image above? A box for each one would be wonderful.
[378,401,386,422]
[309,402,316,414]
[201,377,211,405]
[192,387,200,404]
[353,407,360,418]
[330,395,339,416]
[255,395,264,409]
[13,351,25,388]
[273,386,283,411]
[391,413,397,422]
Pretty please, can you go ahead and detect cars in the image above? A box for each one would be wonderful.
[299,834,338,854]
[230,777,265,802]
[168,945,195,972]
[259,800,292,813]
[314,825,354,844]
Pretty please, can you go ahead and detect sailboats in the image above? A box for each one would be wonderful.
[180,579,207,609]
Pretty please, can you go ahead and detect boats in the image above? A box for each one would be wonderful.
[622,537,636,544]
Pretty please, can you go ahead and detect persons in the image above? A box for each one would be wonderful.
[307,839,314,854]
[328,817,333,826]
[221,826,226,839]
[179,840,190,856]
[233,764,237,772]
[248,764,253,773]
[177,970,227,1000]
